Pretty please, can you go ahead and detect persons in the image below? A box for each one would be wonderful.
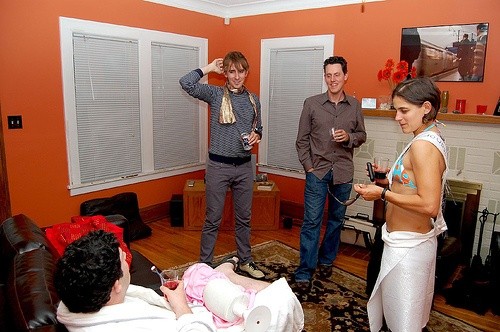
[296,57,367,283]
[457,24,488,81]
[179,51,265,280]
[56,231,306,332]
[353,77,448,331]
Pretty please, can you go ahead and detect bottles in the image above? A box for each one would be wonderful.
[441,90,449,113]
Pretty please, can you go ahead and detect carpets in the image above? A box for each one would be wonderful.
[160,238,483,332]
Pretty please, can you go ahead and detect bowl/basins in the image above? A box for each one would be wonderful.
[256,182,275,191]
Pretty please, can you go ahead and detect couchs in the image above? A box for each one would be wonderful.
[0,212,171,332]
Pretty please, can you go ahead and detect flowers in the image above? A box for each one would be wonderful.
[378,60,416,88]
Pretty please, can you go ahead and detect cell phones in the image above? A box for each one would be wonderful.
[189,181,195,187]
[367,162,375,182]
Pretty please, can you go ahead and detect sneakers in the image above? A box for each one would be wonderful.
[319,264,332,277]
[296,281,311,292]
[239,261,265,278]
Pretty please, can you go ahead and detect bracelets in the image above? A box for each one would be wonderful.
[381,188,392,204]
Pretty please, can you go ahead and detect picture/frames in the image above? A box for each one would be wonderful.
[399,22,489,82]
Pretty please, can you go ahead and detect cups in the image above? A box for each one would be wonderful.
[160,269,179,303]
[379,95,390,110]
[373,157,389,180]
[477,105,488,114]
[328,127,339,141]
[456,99,466,113]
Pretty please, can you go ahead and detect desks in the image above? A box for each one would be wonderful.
[184,178,281,232]
